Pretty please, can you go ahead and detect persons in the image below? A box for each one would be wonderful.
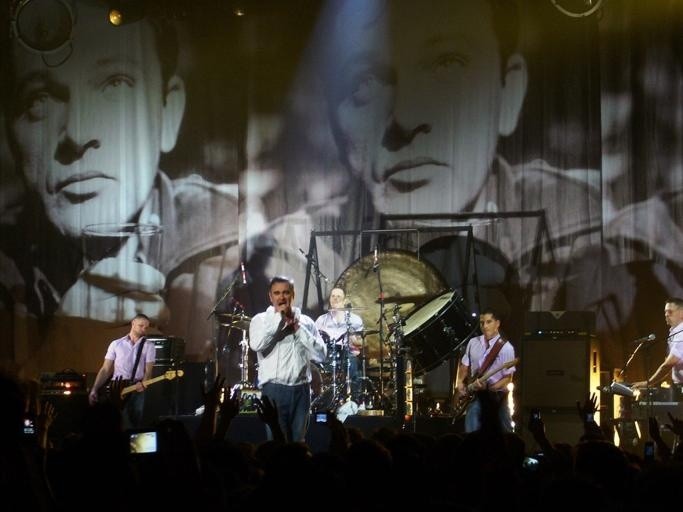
[631,297,683,402]
[0,1,240,363]
[605,189,681,372]
[315,287,364,400]
[455,308,516,434]
[241,1,603,320]
[1,375,681,511]
[89,313,156,429]
[250,276,328,443]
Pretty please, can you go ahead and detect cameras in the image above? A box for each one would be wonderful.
[520,455,541,473]
[122,427,164,458]
[315,411,329,425]
[22,418,42,436]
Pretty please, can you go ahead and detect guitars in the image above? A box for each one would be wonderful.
[97,369,184,409]
[450,357,520,419]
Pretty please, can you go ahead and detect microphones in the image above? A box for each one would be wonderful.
[240,262,248,285]
[396,304,406,326]
[376,308,387,325]
[278,302,286,321]
[374,247,378,265]
[630,333,656,345]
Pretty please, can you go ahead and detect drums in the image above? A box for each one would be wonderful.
[309,361,346,413]
[387,287,481,380]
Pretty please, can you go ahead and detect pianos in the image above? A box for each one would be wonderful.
[630,381,680,412]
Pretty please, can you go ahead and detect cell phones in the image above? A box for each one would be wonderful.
[529,406,541,420]
[644,441,655,461]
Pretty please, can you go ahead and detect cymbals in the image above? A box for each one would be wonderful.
[213,311,252,331]
[349,330,379,336]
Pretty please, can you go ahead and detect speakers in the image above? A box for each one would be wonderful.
[518,335,626,456]
[145,360,217,416]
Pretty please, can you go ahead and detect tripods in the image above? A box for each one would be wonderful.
[229,329,255,400]
[309,324,390,417]
